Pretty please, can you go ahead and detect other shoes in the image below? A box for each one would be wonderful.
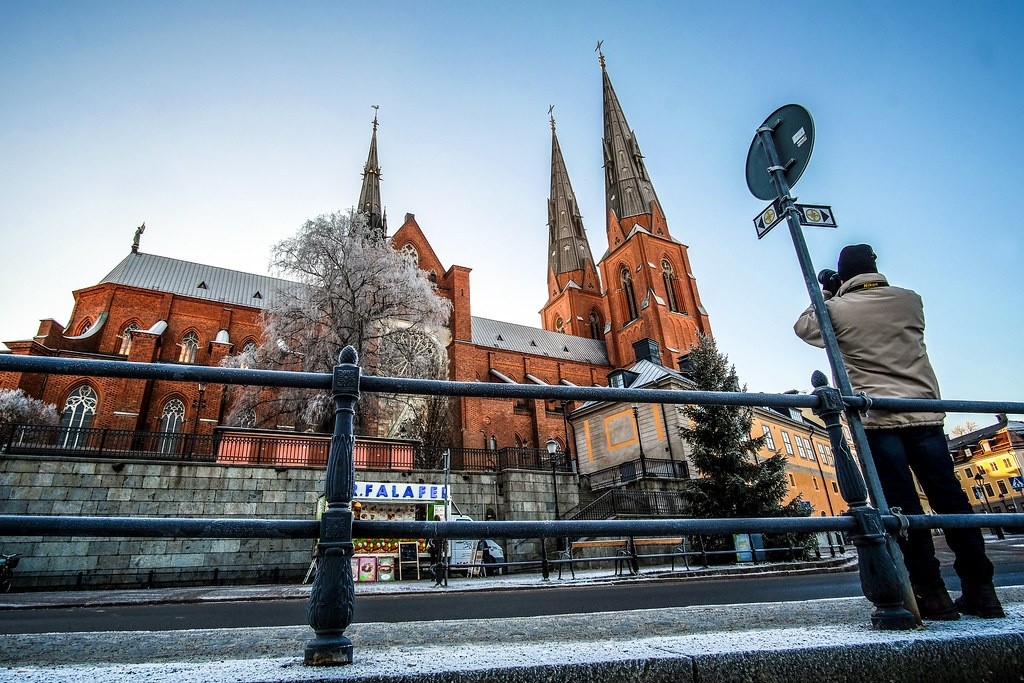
[922,611,960,621]
[955,598,1004,618]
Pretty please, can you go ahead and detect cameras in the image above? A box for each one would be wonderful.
[817,269,831,284]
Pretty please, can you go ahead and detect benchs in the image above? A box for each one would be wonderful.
[551,539,636,577]
[619,538,690,576]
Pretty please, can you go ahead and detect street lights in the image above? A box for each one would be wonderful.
[806,425,847,552]
[546,435,565,551]
[975,472,1006,540]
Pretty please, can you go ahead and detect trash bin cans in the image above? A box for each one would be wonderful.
[730,533,769,562]
[618,460,637,483]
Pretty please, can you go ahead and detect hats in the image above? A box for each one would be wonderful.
[838,244,878,277]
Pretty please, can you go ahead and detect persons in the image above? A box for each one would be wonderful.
[793,244,1006,622]
[133,227,140,246]
[425,515,442,582]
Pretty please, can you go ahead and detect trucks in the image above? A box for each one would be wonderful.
[303,482,506,582]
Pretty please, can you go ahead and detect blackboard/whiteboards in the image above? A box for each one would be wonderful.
[398,541,419,563]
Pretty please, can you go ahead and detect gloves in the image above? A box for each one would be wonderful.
[822,273,838,297]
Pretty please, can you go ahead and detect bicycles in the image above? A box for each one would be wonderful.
[0,552,22,594]
